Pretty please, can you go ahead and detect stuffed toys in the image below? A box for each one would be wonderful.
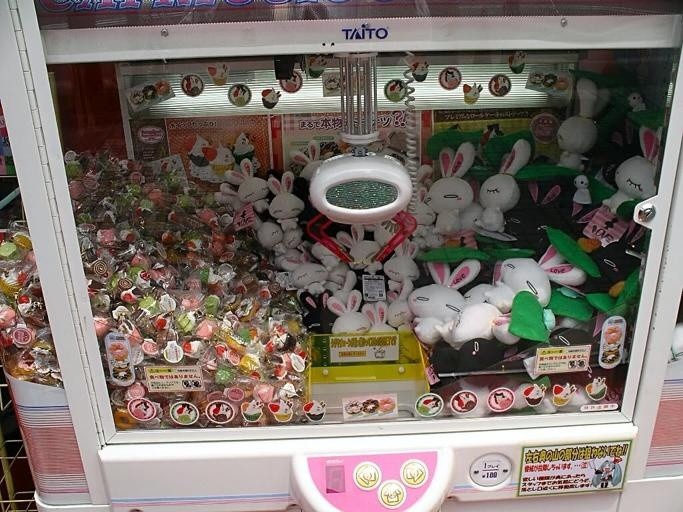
[313,236,346,269]
[284,253,328,295]
[486,244,587,314]
[573,175,592,205]
[225,158,270,212]
[475,139,531,233]
[292,138,324,179]
[216,183,246,211]
[251,212,286,256]
[328,289,370,333]
[410,187,435,236]
[337,224,382,276]
[386,282,415,327]
[408,259,481,344]
[361,302,397,334]
[408,164,433,192]
[269,171,304,231]
[602,126,663,218]
[383,238,419,290]
[426,142,475,236]
[556,78,610,170]
[445,283,520,350]
[628,92,647,111]
